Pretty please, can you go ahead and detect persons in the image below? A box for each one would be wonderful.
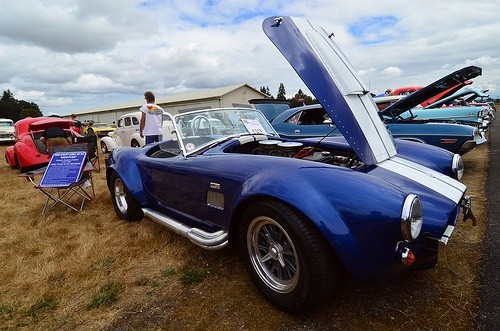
[39,126,72,153]
[295,94,306,107]
[87,120,98,157]
[71,114,81,134]
[139,91,165,144]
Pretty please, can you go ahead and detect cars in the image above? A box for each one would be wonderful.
[80,119,100,126]
[83,123,116,138]
[5,117,85,174]
[106,16,477,313]
[248,66,497,174]
[100,111,183,153]
[0,119,15,143]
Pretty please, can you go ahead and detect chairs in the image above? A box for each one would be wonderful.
[150,148,181,158]
[76,136,101,173]
[18,142,95,214]
